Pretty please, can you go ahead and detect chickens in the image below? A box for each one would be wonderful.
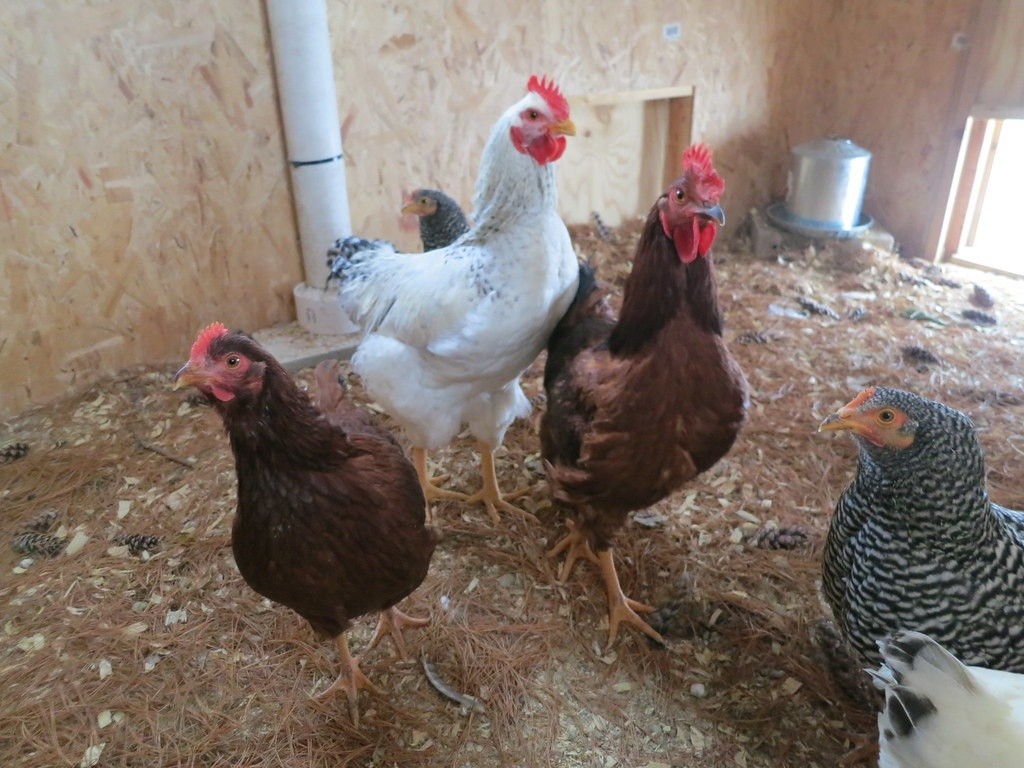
[169,72,753,730]
[814,378,1023,768]
